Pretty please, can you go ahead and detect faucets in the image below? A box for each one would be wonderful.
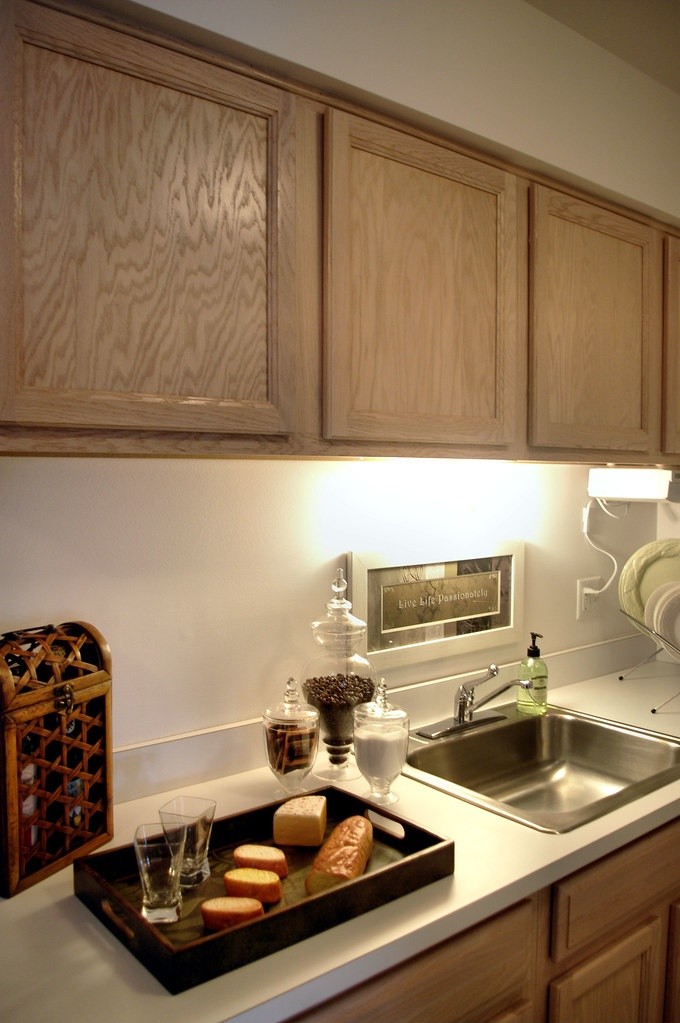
[453,664,535,722]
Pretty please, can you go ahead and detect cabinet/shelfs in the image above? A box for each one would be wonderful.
[278,816,680,1023]
[0,0,679,468]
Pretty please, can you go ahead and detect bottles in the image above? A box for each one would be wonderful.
[351,677,410,805]
[301,568,376,781]
[263,678,320,800]
[12,645,95,856]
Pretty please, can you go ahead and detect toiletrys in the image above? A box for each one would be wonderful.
[515,631,550,715]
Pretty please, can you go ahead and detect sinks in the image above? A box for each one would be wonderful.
[399,697,680,836]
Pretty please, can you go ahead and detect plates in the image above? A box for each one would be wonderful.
[618,538,680,663]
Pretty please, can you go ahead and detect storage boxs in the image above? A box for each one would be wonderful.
[74,785,455,996]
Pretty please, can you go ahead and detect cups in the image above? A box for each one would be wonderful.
[159,796,216,887]
[134,822,187,923]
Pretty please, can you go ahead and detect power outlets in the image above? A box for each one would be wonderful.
[577,577,602,619]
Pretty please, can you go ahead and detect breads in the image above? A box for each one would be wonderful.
[202,795,373,931]
